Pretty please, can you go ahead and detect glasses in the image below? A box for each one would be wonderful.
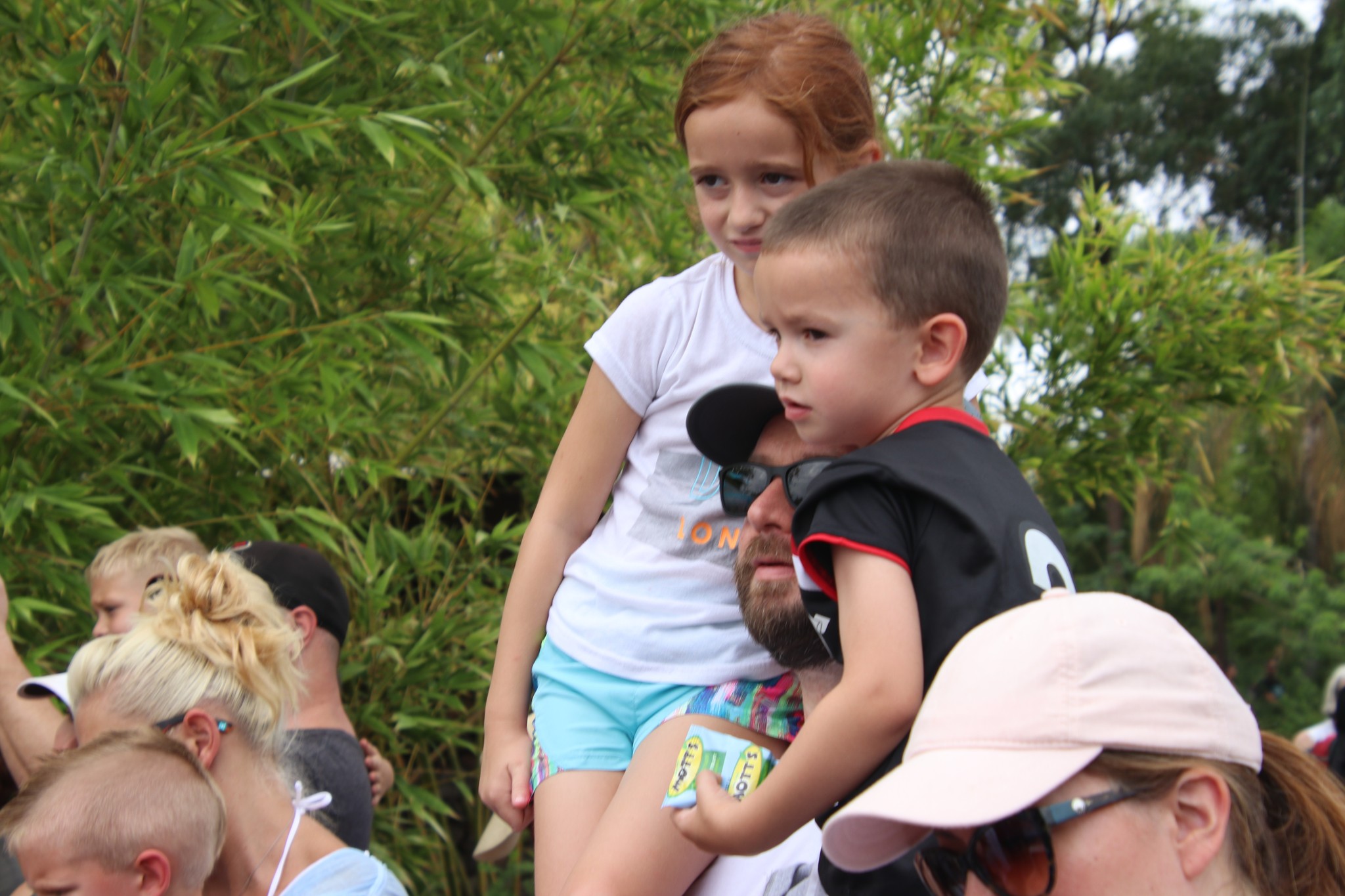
[920,774,1182,896]
[718,458,842,516]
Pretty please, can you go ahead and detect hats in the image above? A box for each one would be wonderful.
[685,383,784,467]
[822,583,1262,872]
[228,539,349,647]
[16,671,77,719]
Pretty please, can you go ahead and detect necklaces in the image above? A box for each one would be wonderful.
[238,811,294,896]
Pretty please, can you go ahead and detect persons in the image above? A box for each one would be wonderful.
[0,524,411,896]
[820,586,1345,896]
[671,155,1076,896]
[681,378,844,896]
[479,11,885,896]
